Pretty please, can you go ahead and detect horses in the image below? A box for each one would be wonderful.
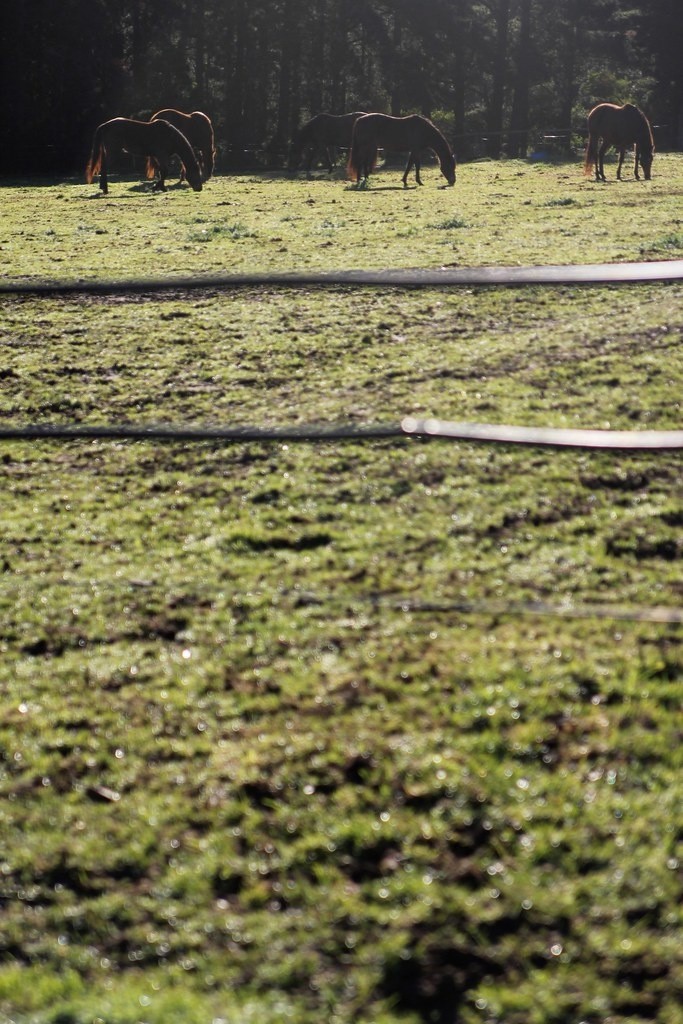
[85,110,216,191]
[581,101,656,181]
[286,111,457,189]
